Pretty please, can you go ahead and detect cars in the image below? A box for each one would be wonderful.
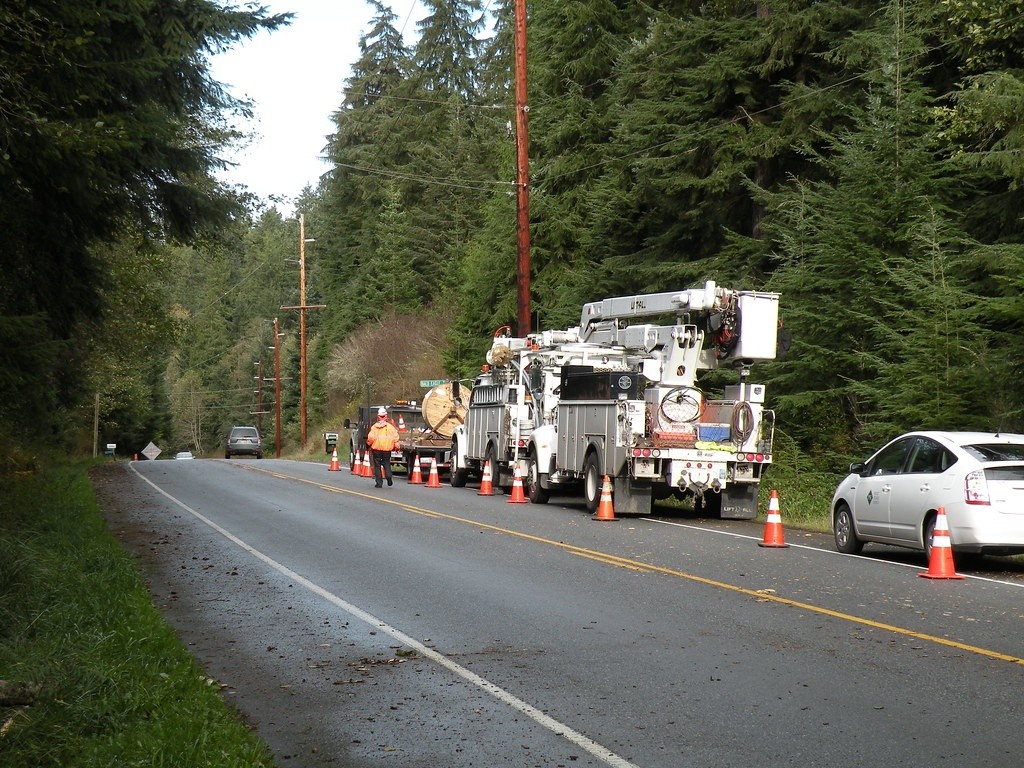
[173,452,195,460]
[830,430,1024,559]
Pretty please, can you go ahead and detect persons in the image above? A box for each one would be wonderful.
[366,408,400,488]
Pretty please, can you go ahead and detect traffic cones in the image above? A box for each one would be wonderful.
[505,462,530,503]
[380,464,386,479]
[360,451,373,477]
[327,447,341,471]
[134,454,138,462]
[424,457,442,488]
[477,460,495,496]
[407,455,426,484]
[591,474,621,521]
[351,450,361,475]
[758,490,791,548]
[916,507,965,579]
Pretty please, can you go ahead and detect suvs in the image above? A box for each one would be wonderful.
[225,425,262,459]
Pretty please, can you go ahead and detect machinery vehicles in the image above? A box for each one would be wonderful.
[344,399,453,482]
[448,279,781,519]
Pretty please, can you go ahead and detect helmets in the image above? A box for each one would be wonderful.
[378,408,387,416]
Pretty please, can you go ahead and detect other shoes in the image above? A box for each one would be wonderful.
[375,485,382,488]
[388,477,392,486]
